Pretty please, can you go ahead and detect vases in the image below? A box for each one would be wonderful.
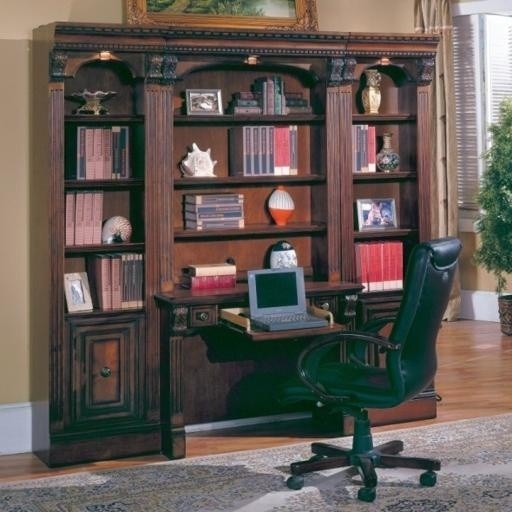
[376,132,400,173]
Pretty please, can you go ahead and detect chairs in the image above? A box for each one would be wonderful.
[286,236,462,501]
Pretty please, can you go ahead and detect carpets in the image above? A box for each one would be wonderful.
[1,411,512,511]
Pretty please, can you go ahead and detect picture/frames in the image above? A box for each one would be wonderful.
[185,88,224,116]
[64,272,94,312]
[356,198,397,231]
[123,1,319,32]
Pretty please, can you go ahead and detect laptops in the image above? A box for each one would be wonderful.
[246,267,328,332]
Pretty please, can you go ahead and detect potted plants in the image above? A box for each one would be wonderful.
[469,96,512,335]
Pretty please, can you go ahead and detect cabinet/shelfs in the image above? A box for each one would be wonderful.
[33,19,441,469]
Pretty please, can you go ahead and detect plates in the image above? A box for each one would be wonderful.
[64,90,118,100]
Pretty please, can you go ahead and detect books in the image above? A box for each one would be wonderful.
[354,242,407,297]
[351,123,380,175]
[180,262,238,279]
[181,191,255,232]
[62,125,146,313]
[222,68,312,177]
[172,275,241,293]
